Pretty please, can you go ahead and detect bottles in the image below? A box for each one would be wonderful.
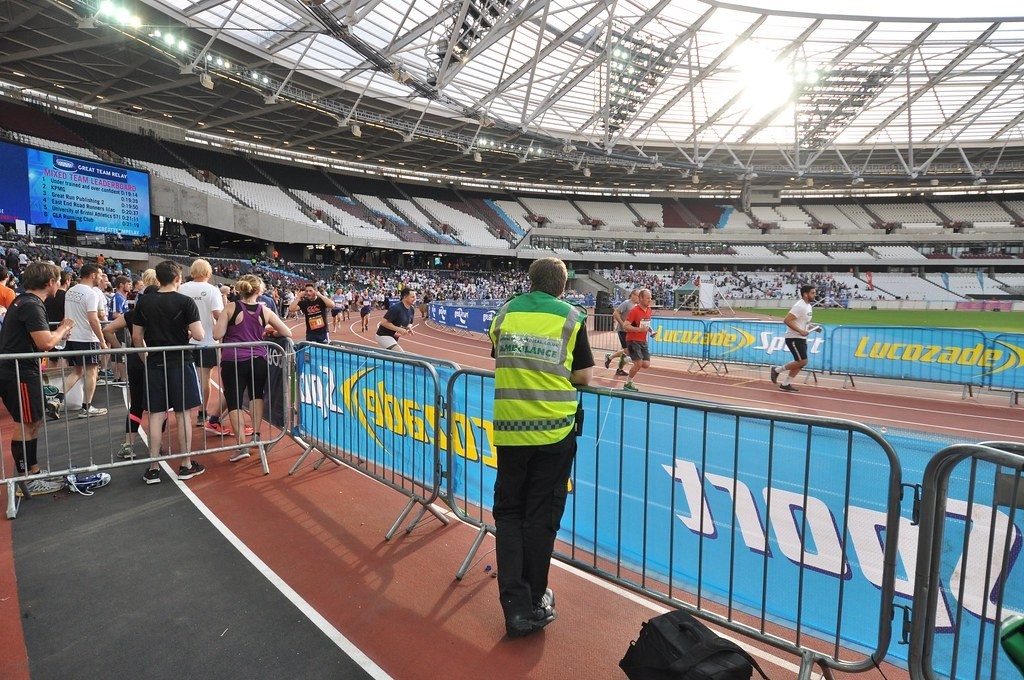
[54,330,68,349]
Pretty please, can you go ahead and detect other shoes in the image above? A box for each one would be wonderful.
[333,329,336,333]
[337,322,340,328]
[362,328,364,333]
[366,325,368,330]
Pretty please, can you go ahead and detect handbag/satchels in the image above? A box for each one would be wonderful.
[619,611,752,680]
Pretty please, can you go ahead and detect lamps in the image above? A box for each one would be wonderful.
[806,178,813,187]
[351,124,361,138]
[474,152,482,163]
[199,70,214,90]
[583,167,591,177]
[692,175,699,184]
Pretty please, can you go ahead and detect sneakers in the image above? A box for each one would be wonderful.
[45,398,61,419]
[37,469,67,481]
[205,418,229,436]
[96,369,131,388]
[619,353,627,370]
[616,369,629,376]
[77,405,108,417]
[623,382,638,392]
[230,424,254,435]
[506,606,557,638]
[229,447,250,462]
[143,466,162,485]
[196,415,211,426]
[115,443,136,458]
[605,354,611,369]
[770,365,779,383]
[251,434,261,444]
[179,461,205,479]
[16,481,65,496]
[533,588,555,608]
[780,384,800,392]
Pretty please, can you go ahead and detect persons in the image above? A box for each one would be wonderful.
[771,286,822,393]
[70,252,160,388]
[604,289,657,392]
[216,249,335,297]
[563,288,593,300]
[102,285,168,459]
[487,256,595,636]
[45,264,108,419]
[131,261,205,484]
[177,258,224,426]
[330,267,402,333]
[374,288,416,353]
[288,283,335,344]
[0,262,76,496]
[607,267,771,305]
[115,230,185,254]
[400,259,532,320]
[776,269,868,298]
[0,223,72,369]
[214,274,292,461]
